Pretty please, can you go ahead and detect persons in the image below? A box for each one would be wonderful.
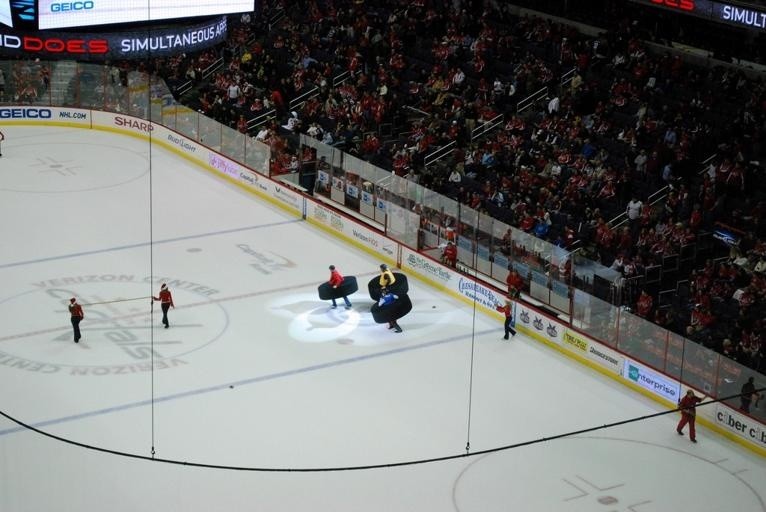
[1,1,766,422]
[678,390,708,444]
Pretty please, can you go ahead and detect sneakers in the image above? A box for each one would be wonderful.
[691,438,696,442]
[75,335,81,343]
[162,320,169,328]
[504,331,516,339]
[677,429,683,435]
[332,304,351,309]
[388,326,402,332]
